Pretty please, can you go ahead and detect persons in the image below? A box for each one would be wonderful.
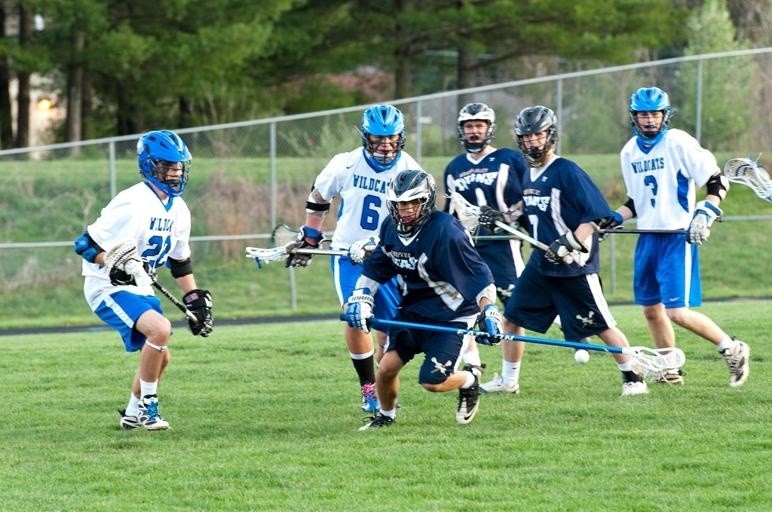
[284,105,423,413]
[480,105,649,396]
[746,160,772,181]
[75,130,214,430]
[443,102,590,367]
[345,169,506,428]
[598,88,750,388]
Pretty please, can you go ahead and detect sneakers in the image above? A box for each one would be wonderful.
[658,368,684,387]
[719,339,749,387]
[137,394,169,431]
[478,374,518,393]
[357,413,396,430]
[119,414,140,429]
[621,381,649,396]
[361,384,380,412]
[455,366,480,426]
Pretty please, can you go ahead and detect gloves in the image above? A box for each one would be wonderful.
[285,230,322,268]
[477,206,506,234]
[688,201,725,244]
[344,289,375,334]
[107,256,136,287]
[545,231,586,266]
[595,211,623,241]
[183,289,213,338]
[475,305,505,347]
[349,238,380,264]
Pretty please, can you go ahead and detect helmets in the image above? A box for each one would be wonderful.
[627,87,671,140]
[358,106,406,172]
[385,168,436,234]
[513,106,560,168]
[137,132,193,198]
[455,103,497,151]
